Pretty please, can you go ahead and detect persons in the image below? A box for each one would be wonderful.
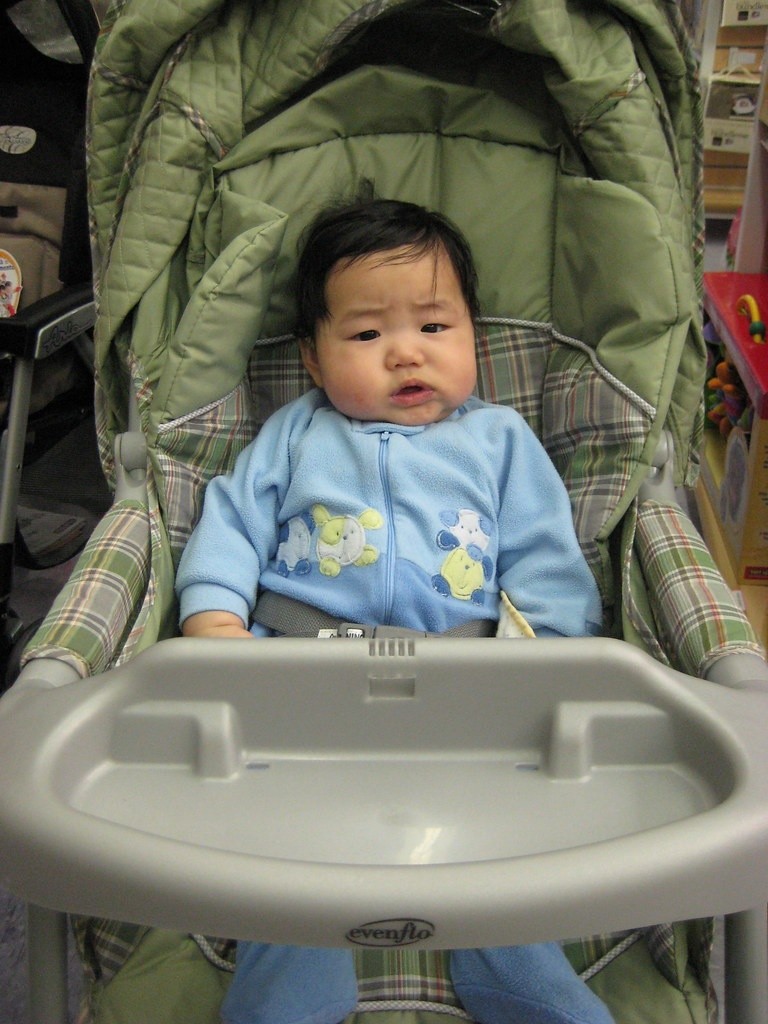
[174,199,614,1024]
[0,281,22,316]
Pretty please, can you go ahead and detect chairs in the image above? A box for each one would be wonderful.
[0,0,100,639]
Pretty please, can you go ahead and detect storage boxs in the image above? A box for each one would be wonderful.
[695,273,768,588]
